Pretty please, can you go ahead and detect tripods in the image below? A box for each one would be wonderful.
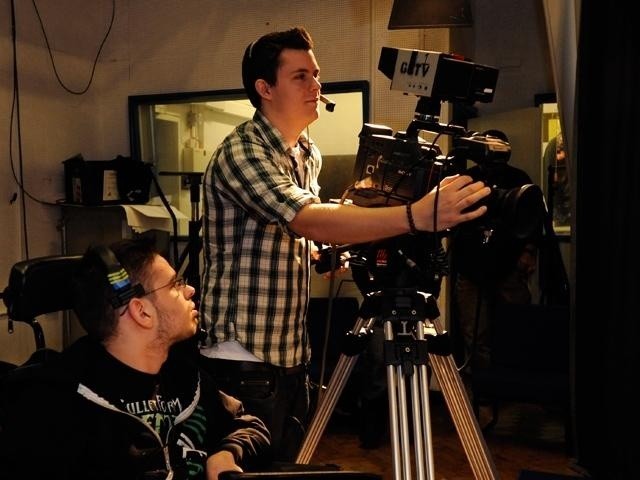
[294,294,499,480]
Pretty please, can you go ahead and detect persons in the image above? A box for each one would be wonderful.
[197,26,492,466]
[449,128,544,430]
[25,238,272,480]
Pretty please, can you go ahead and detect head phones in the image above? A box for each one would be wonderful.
[95,243,146,309]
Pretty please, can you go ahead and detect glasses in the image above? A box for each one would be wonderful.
[120,278,187,317]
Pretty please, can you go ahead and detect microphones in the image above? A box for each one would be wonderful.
[320,94,336,112]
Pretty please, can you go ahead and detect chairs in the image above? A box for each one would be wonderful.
[0,255,81,350]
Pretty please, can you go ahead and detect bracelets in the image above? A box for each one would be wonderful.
[407,201,417,233]
